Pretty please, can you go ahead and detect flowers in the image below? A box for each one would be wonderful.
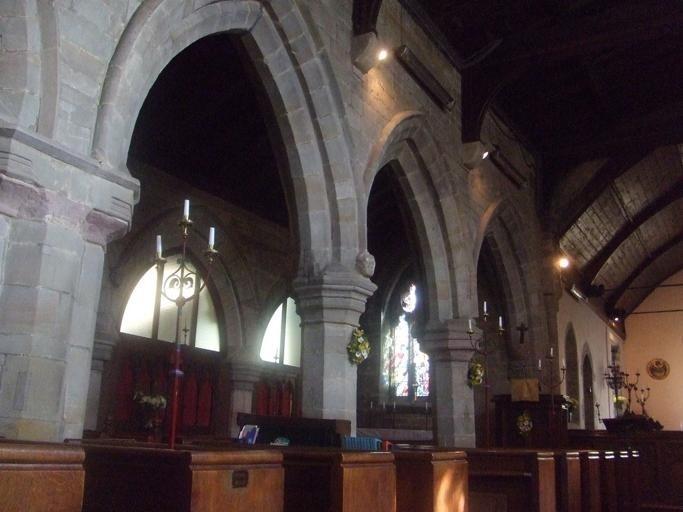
[613,396,626,410]
[516,410,534,435]
[469,363,484,386]
[132,390,167,409]
[346,327,372,365]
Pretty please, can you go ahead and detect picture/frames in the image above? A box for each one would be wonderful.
[237,424,259,445]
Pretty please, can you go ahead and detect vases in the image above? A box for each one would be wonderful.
[141,405,160,439]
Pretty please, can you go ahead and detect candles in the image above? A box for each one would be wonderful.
[468,320,471,331]
[208,227,215,248]
[184,199,189,220]
[499,316,502,328]
[563,359,565,368]
[156,235,162,257]
[538,359,541,367]
[483,301,486,314]
[550,347,553,356]
